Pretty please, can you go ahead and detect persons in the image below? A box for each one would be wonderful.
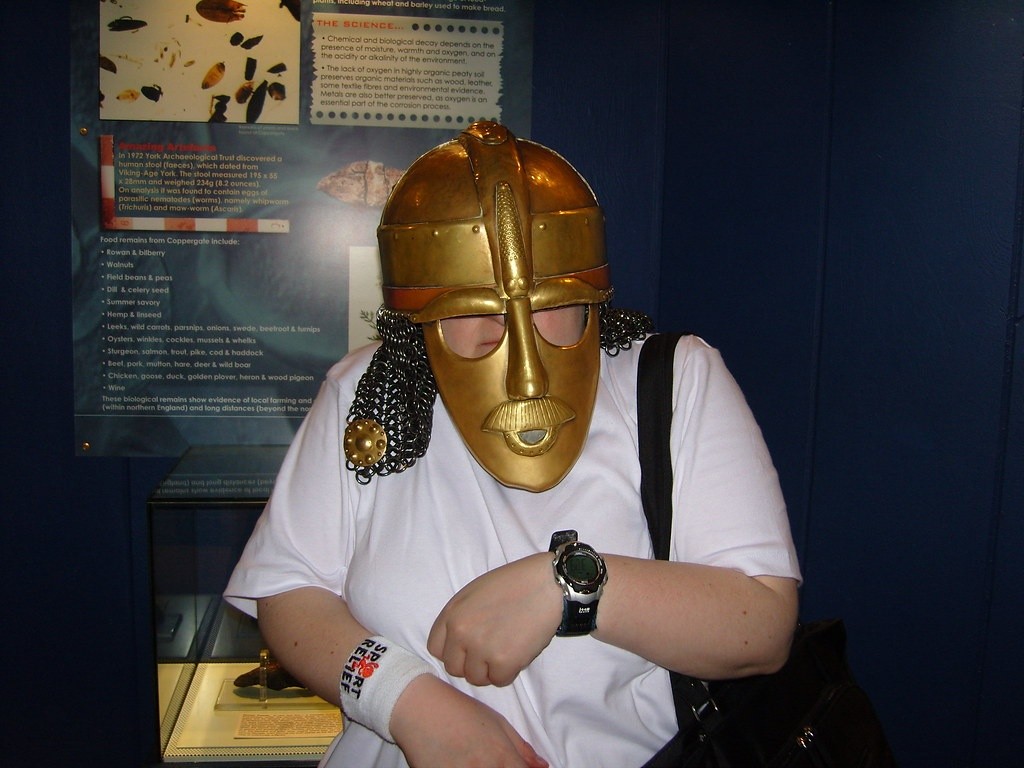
[222,119,803,768]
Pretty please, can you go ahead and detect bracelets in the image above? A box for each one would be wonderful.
[340,635,438,743]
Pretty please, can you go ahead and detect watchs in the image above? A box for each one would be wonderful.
[549,529,608,638]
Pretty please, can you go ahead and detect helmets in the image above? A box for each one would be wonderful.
[342,121,652,494]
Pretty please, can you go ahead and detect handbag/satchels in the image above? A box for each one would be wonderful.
[634,330,896,767]
